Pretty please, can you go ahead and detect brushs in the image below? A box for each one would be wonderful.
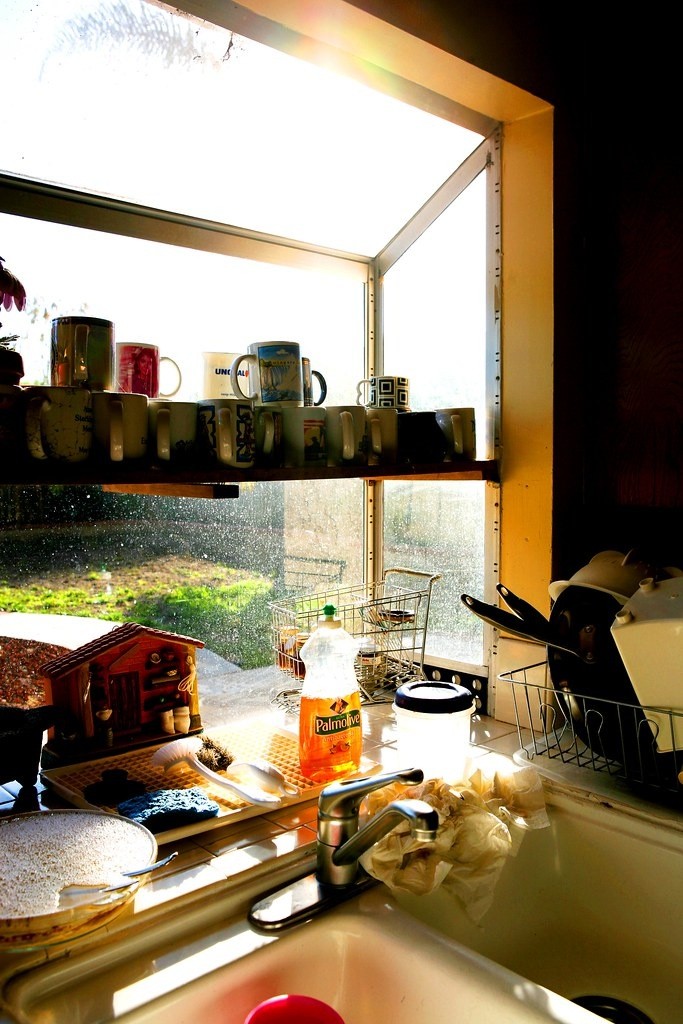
[150,737,283,809]
[227,756,303,799]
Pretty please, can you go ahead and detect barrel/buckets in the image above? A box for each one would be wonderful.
[393,681,476,785]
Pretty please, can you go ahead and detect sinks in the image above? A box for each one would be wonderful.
[378,808,683,1024]
[100,913,567,1024]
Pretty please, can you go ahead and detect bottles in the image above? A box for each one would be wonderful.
[276,625,385,681]
[94,722,114,750]
[299,605,362,779]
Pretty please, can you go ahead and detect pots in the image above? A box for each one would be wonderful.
[462,583,683,783]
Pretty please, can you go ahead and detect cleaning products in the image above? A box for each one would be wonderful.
[298,604,364,784]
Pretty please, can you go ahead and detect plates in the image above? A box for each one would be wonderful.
[571,994,654,1023]
[378,609,416,617]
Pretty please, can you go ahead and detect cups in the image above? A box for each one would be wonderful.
[436,408,476,462]
[202,350,248,399]
[253,405,305,468]
[149,401,199,461]
[0,315,181,462]
[301,357,326,407]
[397,412,446,466]
[357,377,409,412]
[243,994,346,1024]
[365,407,398,466]
[325,406,366,467]
[304,408,328,467]
[198,399,255,468]
[232,341,305,407]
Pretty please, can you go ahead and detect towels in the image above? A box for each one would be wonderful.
[117,786,220,834]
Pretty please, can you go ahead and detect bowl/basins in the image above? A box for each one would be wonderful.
[0,809,159,953]
[547,549,650,605]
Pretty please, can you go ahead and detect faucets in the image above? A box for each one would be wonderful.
[316,766,439,888]
